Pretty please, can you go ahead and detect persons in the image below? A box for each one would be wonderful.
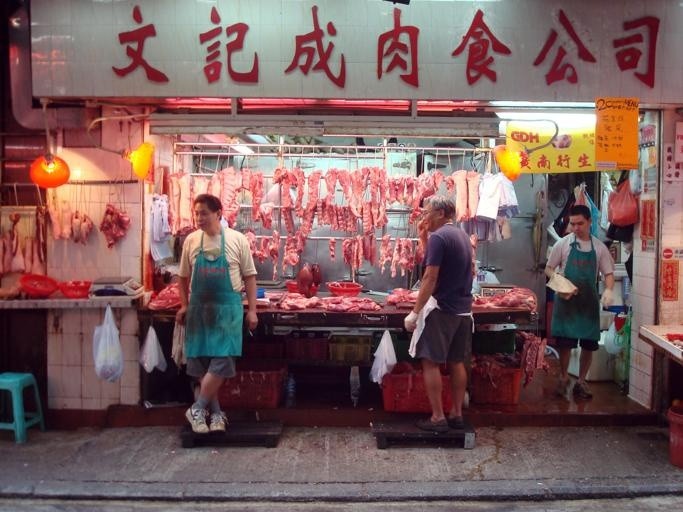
[543,204,616,401]
[403,195,474,433]
[175,194,259,434]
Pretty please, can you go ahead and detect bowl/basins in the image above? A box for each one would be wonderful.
[18,273,58,298]
[326,280,363,296]
[256,287,265,299]
[286,279,320,297]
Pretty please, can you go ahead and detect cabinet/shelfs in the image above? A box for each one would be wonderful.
[174,142,490,246]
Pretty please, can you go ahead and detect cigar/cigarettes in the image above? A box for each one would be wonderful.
[248,330,253,337]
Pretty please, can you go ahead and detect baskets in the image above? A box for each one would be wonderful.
[21,274,59,299]
[285,282,320,296]
[327,282,363,297]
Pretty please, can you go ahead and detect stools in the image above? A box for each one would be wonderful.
[1,372,45,445]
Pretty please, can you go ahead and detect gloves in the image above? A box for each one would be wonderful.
[601,289,613,309]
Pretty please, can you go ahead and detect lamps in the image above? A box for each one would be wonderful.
[86,106,154,182]
[29,99,70,188]
[492,119,558,181]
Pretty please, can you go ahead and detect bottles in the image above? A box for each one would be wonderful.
[284,373,297,409]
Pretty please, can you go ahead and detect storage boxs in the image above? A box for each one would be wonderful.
[212,362,291,413]
[564,303,629,382]
[384,360,454,415]
[469,367,527,406]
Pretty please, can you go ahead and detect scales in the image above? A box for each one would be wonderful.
[91,276,145,296]
[479,283,517,297]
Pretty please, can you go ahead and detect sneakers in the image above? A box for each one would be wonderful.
[446,416,465,429]
[184,404,209,434]
[573,382,592,400]
[210,411,229,433]
[557,379,572,398]
[416,416,449,432]
